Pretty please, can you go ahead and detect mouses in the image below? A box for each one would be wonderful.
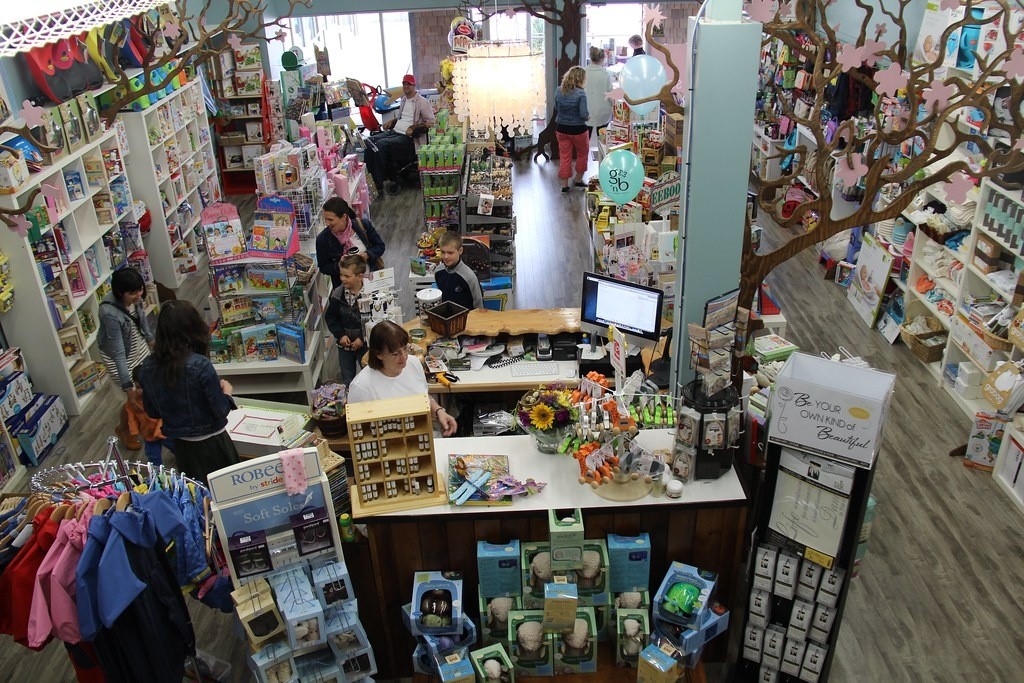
[565,368,576,378]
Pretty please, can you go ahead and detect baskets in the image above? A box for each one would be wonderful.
[899,299,944,349]
[982,330,1014,352]
[910,330,949,363]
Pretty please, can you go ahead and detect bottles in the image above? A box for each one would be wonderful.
[339,513,355,543]
[416,288,443,326]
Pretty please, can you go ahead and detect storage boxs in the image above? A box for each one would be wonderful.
[19,394,71,465]
[75,375,103,398]
[12,392,48,459]
[59,325,85,352]
[39,261,54,282]
[72,360,97,385]
[415,113,465,218]
[0,149,31,194]
[588,97,686,273]
[200,202,249,265]
[82,309,95,333]
[77,311,90,339]
[229,507,733,683]
[245,192,301,260]
[208,42,373,213]
[424,300,471,335]
[61,332,86,361]
[208,266,315,365]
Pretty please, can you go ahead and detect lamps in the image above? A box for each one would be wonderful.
[452,0,547,141]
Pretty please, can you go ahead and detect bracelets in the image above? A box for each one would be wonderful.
[435,407,446,416]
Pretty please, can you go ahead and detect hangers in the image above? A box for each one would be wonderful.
[0,456,214,557]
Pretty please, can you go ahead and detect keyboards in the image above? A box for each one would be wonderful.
[511,362,559,376]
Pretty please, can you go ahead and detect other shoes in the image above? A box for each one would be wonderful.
[560,184,572,194]
[573,180,588,188]
[114,424,142,451]
[373,191,384,202]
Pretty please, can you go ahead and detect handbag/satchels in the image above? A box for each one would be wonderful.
[368,244,385,269]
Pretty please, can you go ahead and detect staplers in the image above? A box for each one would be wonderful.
[536,333,553,361]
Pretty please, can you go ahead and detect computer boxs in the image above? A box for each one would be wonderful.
[577,350,645,379]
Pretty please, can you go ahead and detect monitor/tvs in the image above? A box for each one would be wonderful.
[581,271,664,356]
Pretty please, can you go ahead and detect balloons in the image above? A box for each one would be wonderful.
[598,148,645,205]
[619,54,667,116]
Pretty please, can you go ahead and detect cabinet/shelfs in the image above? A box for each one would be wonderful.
[115,73,228,291]
[345,392,442,517]
[758,29,1024,430]
[0,100,163,417]
[351,430,749,677]
[467,154,518,290]
[215,69,267,196]
[206,267,334,407]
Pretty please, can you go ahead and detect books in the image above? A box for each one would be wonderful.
[205,219,247,260]
[251,209,292,252]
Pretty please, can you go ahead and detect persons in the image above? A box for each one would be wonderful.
[860,265,880,300]
[553,67,591,195]
[98,268,163,467]
[348,321,457,437]
[923,35,936,61]
[583,46,613,140]
[325,255,370,384]
[987,429,1004,463]
[630,35,645,56]
[435,231,484,310]
[316,197,386,288]
[363,75,436,175]
[131,301,238,490]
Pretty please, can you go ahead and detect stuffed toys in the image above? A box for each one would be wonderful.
[484,517,649,683]
[293,618,320,644]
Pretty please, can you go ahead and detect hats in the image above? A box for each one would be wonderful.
[402,74,416,85]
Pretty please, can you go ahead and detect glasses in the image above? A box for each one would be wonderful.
[379,345,411,359]
